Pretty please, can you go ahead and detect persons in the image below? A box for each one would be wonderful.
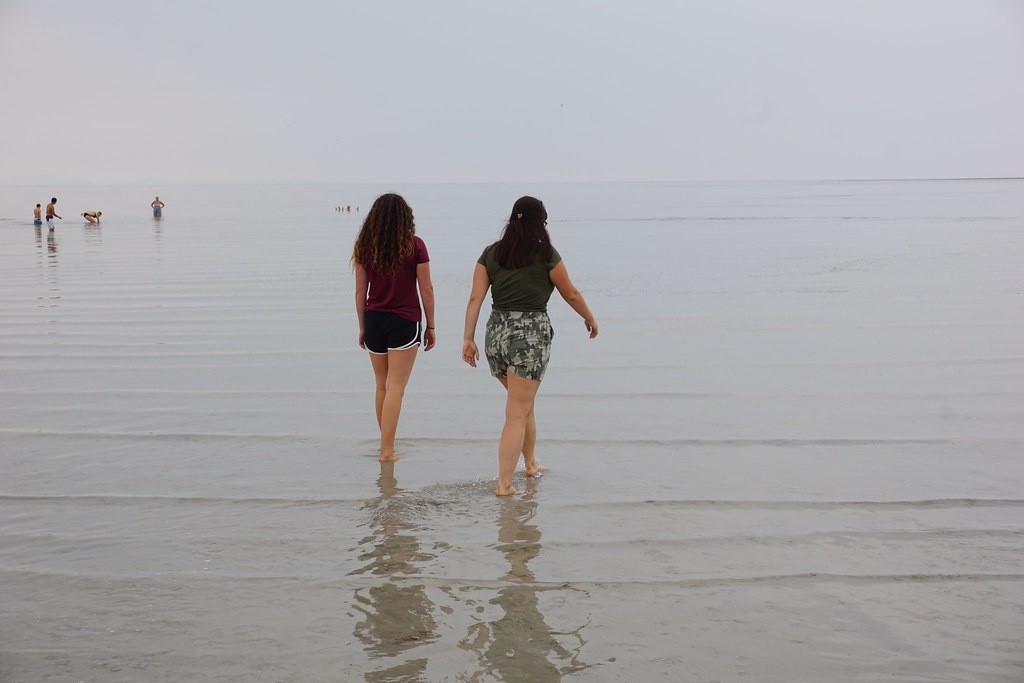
[151,196,165,217]
[350,193,436,463]
[81,209,102,224]
[46,198,62,232]
[34,203,42,224]
[462,197,599,496]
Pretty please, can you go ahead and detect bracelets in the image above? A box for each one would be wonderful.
[426,326,436,330]
